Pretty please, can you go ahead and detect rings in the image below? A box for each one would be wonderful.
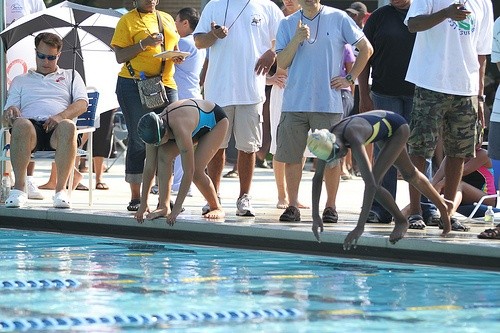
[264,65,268,69]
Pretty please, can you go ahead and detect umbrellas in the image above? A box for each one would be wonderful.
[0,0,125,120]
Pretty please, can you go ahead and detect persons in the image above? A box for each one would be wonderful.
[2,33,89,208]
[23,0,500,251]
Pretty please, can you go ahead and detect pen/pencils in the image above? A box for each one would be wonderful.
[144,30,164,46]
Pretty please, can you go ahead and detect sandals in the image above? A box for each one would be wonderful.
[477,223,500,239]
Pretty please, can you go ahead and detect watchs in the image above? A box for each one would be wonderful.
[345,73,355,85]
[477,95,486,103]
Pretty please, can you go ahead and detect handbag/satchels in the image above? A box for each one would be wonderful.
[137,76,170,110]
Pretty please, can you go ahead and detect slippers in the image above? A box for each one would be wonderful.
[52,190,70,207]
[5,190,28,208]
[66,183,89,191]
[96,182,109,190]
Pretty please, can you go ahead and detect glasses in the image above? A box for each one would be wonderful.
[326,144,340,163]
[154,121,162,145]
[36,50,58,60]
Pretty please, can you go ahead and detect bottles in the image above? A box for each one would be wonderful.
[2,173,11,203]
[485,206,494,230]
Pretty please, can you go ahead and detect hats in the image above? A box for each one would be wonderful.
[345,2,367,14]
[307,129,332,161]
[137,112,165,144]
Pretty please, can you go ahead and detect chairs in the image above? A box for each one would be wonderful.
[0,84,101,208]
[106,108,128,171]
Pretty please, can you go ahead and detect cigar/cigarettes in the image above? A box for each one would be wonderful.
[46,125,48,130]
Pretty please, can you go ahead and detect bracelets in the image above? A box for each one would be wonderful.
[139,39,146,51]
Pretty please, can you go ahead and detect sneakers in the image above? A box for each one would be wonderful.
[27,182,45,199]
[236,193,255,217]
[202,193,222,215]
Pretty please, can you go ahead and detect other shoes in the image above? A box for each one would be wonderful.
[438,216,470,232]
[423,210,440,226]
[407,215,426,229]
[157,200,185,212]
[222,169,238,178]
[322,207,338,223]
[127,199,140,211]
[278,206,300,222]
[366,212,380,223]
